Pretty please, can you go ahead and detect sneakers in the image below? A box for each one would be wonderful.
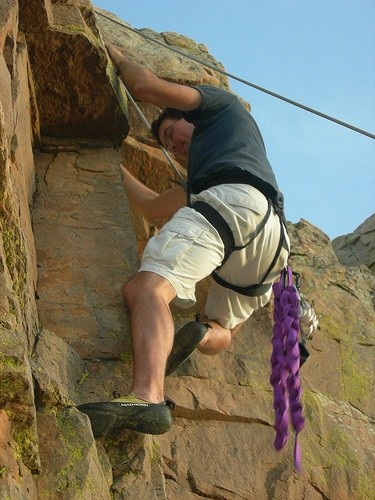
[77,390,175,438]
[165,313,213,377]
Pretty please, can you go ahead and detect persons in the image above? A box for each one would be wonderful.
[76,43,290,435]
